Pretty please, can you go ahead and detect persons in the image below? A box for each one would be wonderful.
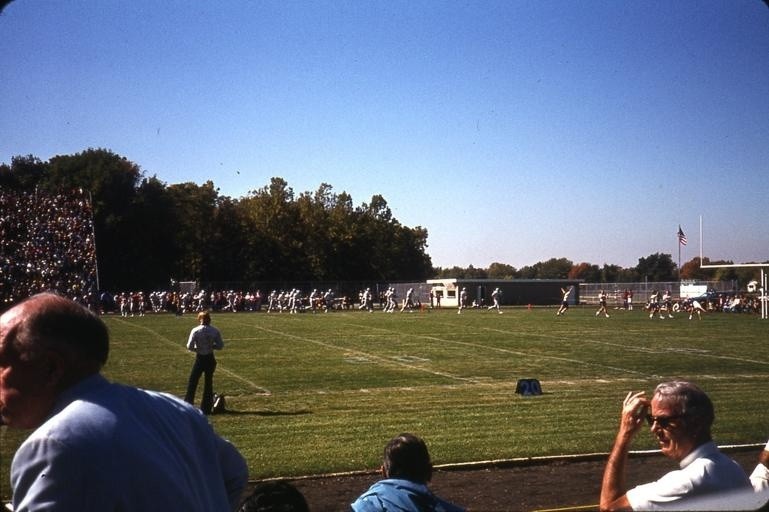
[358,286,420,313]
[458,287,469,314]
[100,291,263,318]
[1,187,102,316]
[429,290,440,307]
[596,289,610,318]
[750,440,769,511]
[599,379,756,512]
[0,291,250,512]
[487,288,504,315]
[267,288,354,315]
[350,432,466,511]
[613,285,762,320]
[557,287,573,317]
[184,309,224,415]
[236,479,309,512]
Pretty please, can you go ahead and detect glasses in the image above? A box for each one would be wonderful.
[646,413,683,426]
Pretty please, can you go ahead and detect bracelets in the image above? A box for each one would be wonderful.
[764,440,769,450]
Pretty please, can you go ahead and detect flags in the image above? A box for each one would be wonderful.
[678,227,688,246]
